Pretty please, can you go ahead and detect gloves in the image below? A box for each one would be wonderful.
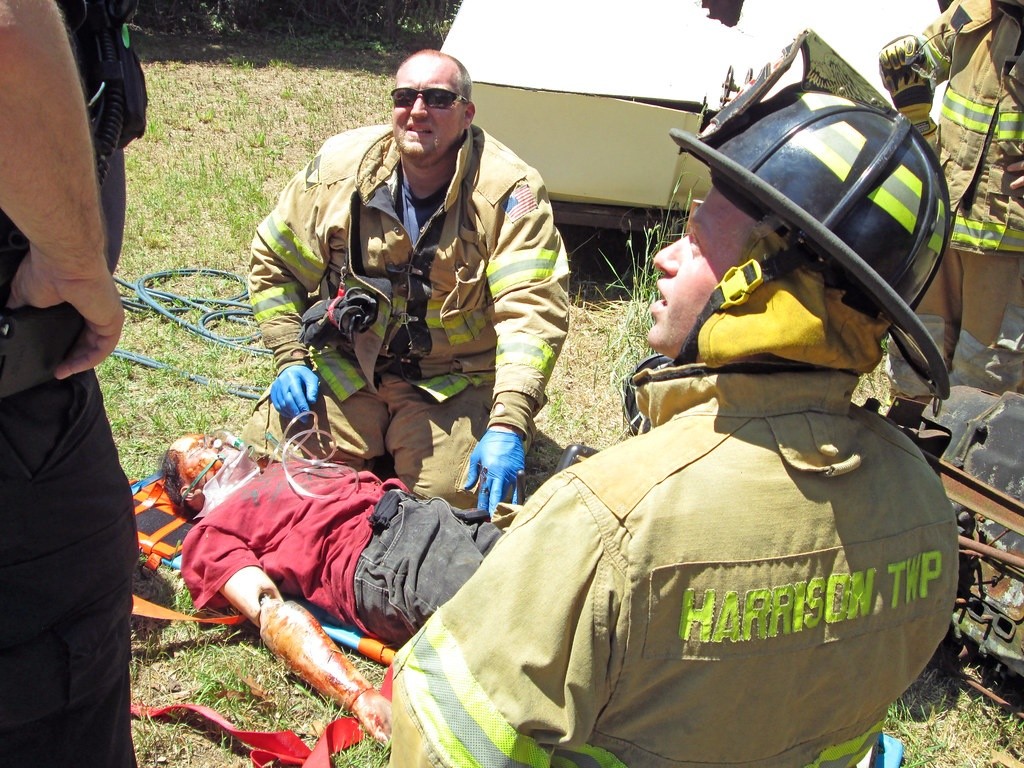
[270,366,319,423]
[882,56,937,139]
[879,34,934,90]
[465,429,525,519]
[298,287,379,351]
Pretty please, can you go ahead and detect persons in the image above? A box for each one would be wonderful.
[0,0,152,768]
[248,49,570,518]
[388,95,961,768]
[163,435,507,748]
[878,0,1024,425]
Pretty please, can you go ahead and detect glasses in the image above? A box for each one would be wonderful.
[391,87,469,109]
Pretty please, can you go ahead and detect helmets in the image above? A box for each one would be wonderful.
[668,27,951,419]
